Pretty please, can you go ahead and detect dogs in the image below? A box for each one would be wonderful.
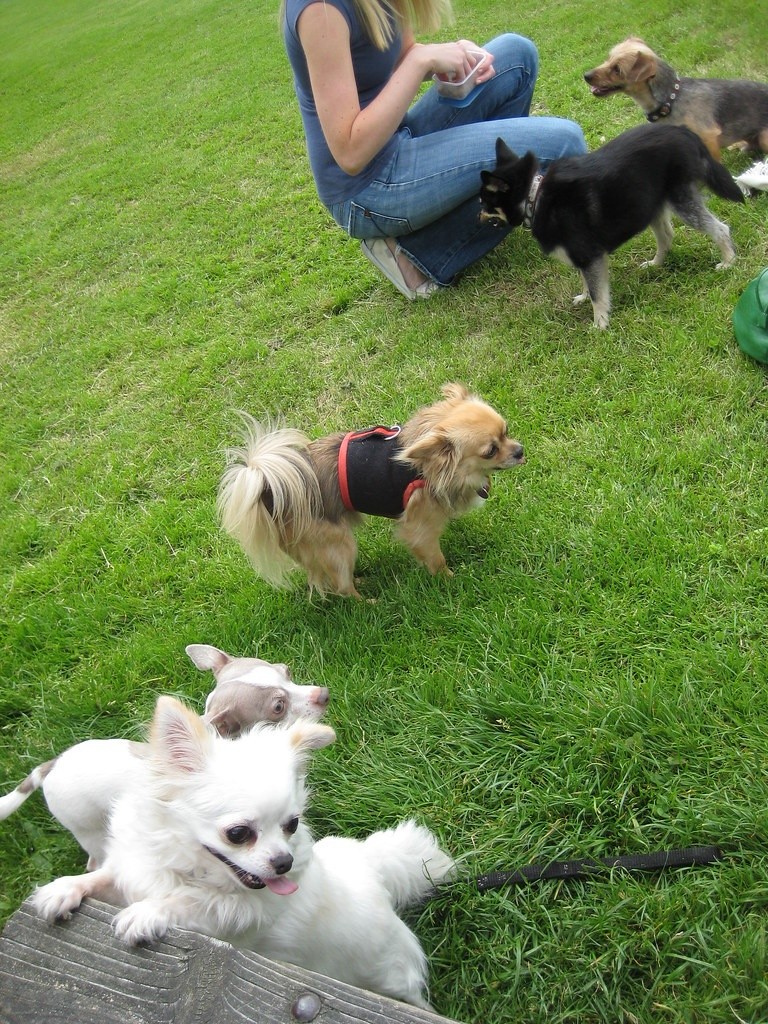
[478,36,768,332]
[215,379,528,612]
[0,643,462,1016]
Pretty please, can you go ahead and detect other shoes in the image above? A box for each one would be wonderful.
[360,238,438,302]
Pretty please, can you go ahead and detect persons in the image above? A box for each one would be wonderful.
[278,0,587,300]
